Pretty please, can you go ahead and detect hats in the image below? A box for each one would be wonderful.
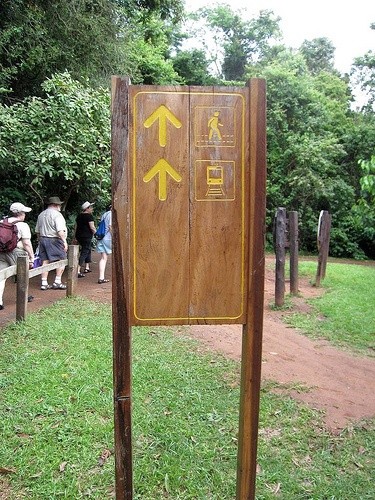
[10,202,32,213]
[45,197,64,204]
[82,201,95,212]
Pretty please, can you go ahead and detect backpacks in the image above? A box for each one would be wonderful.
[0,218,24,252]
[94,212,111,240]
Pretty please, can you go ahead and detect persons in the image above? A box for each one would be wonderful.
[0,202,34,309]
[97,203,113,284]
[73,201,97,277]
[34,196,69,290]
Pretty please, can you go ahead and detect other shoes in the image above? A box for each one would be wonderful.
[84,269,91,272]
[0,305,4,310]
[98,279,109,283]
[78,273,84,277]
[28,296,33,302]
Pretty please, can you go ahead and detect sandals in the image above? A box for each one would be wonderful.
[41,284,52,289]
[52,282,67,289]
